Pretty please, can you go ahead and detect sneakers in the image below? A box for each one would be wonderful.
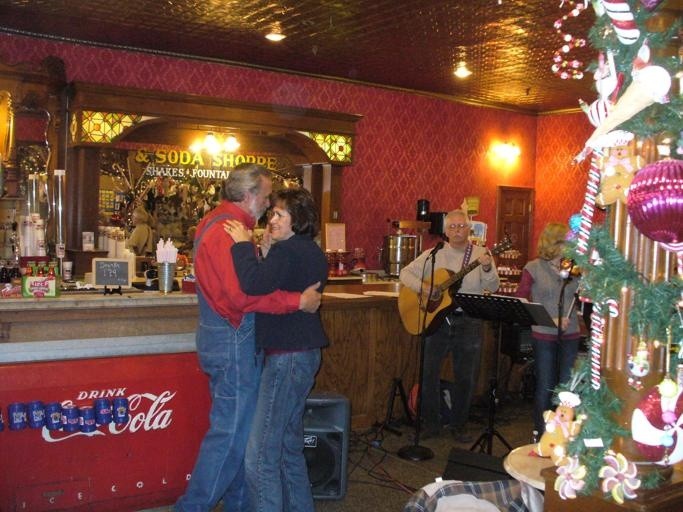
[408,422,443,441]
[449,422,474,443]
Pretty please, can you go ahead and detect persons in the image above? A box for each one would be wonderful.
[126,207,156,256]
[513,223,582,442]
[398,208,500,443]
[173,163,330,511]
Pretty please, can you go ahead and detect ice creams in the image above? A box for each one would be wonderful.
[585,65,672,148]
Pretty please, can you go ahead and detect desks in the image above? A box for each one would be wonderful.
[540,481,683,512]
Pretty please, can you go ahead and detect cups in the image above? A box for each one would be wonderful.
[63,262,73,279]
[157,262,176,293]
[99,226,125,258]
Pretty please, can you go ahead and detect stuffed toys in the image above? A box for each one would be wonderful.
[532,391,582,468]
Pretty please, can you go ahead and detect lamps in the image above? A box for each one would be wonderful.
[189,131,241,156]
[264,25,287,41]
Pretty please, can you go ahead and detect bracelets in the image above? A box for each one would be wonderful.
[483,265,491,273]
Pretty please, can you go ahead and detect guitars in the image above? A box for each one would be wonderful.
[398,238,516,336]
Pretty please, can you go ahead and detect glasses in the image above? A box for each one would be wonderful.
[447,222,467,230]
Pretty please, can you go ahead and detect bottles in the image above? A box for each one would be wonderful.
[25,262,59,277]
[326,248,346,277]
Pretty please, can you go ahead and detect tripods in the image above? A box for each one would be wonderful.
[469,320,514,455]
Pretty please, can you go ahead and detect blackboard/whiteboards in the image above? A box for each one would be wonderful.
[92,257,132,290]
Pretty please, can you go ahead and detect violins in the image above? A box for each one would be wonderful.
[563,259,591,277]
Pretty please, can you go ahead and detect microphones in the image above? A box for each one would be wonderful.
[426,241,445,260]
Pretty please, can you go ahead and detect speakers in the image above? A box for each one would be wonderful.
[442,446,512,481]
[303,392,351,501]
